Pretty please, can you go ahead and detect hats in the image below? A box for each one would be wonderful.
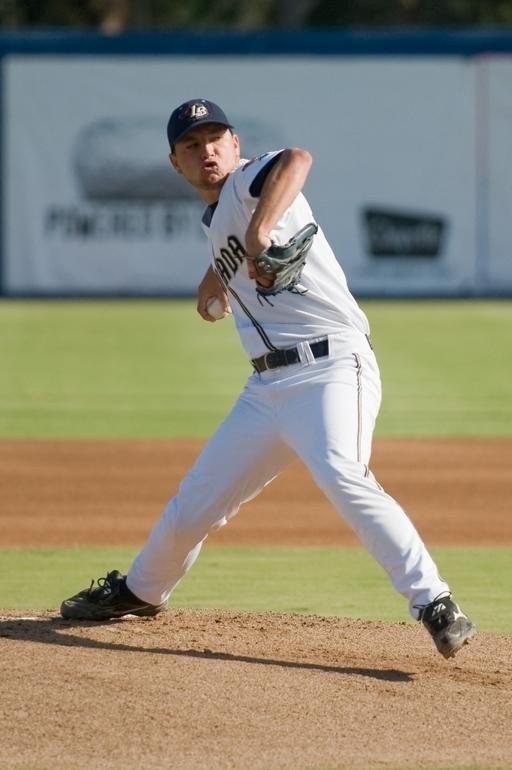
[166,98,236,150]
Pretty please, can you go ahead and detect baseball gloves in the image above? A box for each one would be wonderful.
[255,223,317,296]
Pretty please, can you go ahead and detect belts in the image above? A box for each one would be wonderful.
[248,333,376,375]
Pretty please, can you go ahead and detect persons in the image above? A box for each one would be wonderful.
[61,100,476,659]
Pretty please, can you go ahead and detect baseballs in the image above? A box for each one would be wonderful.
[206,296,228,320]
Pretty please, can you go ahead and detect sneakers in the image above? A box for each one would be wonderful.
[420,594,477,661]
[60,570,168,622]
